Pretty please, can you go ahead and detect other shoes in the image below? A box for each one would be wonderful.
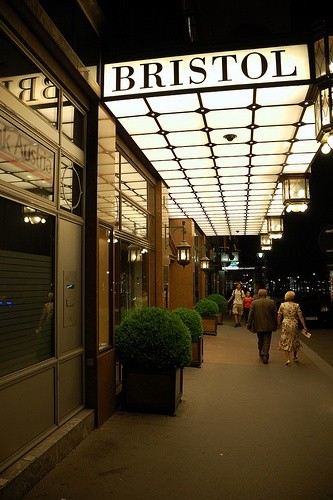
[285,360,290,366]
[263,360,268,364]
[235,323,241,327]
[293,357,297,362]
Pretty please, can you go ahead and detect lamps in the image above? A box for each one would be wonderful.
[313,32,333,154]
[19,186,53,224]
[259,234,272,251]
[162,221,191,269]
[127,243,144,266]
[265,216,284,239]
[280,173,311,212]
[201,255,210,272]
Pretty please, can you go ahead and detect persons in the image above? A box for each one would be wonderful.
[228,283,259,327]
[247,289,277,364]
[277,291,307,364]
[37,292,54,330]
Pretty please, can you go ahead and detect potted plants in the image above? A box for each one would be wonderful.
[208,293,228,325]
[172,307,204,367]
[116,305,193,417]
[193,297,219,337]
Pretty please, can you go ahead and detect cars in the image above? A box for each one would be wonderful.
[296,292,332,328]
